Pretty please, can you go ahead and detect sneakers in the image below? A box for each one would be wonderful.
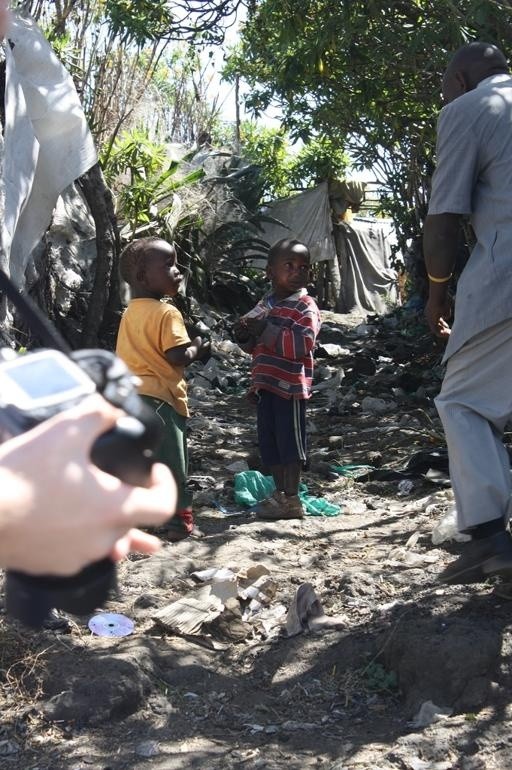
[255,492,307,518]
[168,509,193,540]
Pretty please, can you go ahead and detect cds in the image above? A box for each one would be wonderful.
[88,613,135,638]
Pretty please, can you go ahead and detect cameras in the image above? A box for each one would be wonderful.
[0,346,166,626]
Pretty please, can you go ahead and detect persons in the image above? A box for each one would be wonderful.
[230,238,321,518]
[116,237,210,540]
[423,43,512,582]
[0,407,178,576]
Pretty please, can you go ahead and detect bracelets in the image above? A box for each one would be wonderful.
[428,273,453,283]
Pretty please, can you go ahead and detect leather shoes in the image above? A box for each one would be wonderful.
[441,531,512,584]
[495,580,512,600]
